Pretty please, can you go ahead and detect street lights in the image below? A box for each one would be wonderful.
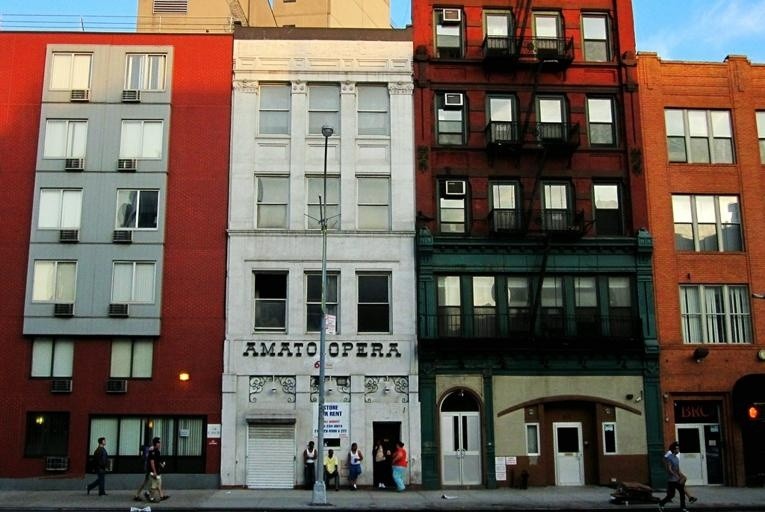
[308,122,343,503]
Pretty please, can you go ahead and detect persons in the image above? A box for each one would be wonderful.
[304,441,317,490]
[323,449,339,491]
[375,439,392,488]
[149,440,169,503]
[664,442,697,504]
[348,443,363,489]
[659,444,689,512]
[392,441,407,491]
[134,438,160,501]
[87,438,108,496]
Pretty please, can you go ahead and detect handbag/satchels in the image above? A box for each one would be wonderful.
[375,445,385,462]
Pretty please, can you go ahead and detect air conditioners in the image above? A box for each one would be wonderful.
[54,303,75,317]
[71,89,90,102]
[65,159,85,170]
[59,230,79,243]
[440,9,461,23]
[51,380,72,392]
[112,230,133,243]
[443,93,463,107]
[107,380,128,393]
[108,304,129,317]
[122,90,141,102]
[46,457,69,471]
[446,180,466,196]
[117,159,138,170]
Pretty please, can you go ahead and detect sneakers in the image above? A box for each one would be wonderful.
[680,507,689,512]
[659,500,665,512]
[135,492,170,503]
